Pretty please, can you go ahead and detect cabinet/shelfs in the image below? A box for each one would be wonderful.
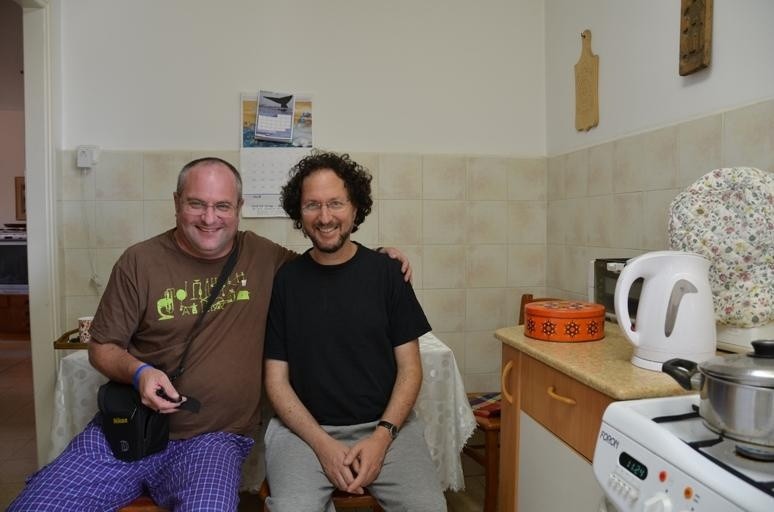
[497,342,617,512]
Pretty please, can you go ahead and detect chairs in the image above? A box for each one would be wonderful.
[465,290,561,511]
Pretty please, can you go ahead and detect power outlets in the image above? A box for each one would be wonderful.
[78,146,99,168]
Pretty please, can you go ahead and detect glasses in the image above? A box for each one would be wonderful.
[303,200,349,216]
[179,199,238,221]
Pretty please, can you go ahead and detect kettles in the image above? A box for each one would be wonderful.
[614,250,717,373]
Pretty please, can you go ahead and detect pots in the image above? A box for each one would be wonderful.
[662,339,773,458]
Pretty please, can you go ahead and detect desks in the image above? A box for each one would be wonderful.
[45,328,474,491]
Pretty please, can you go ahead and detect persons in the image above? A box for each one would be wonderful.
[5,157,413,512]
[263,150,448,511]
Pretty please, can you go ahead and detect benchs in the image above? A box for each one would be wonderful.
[119,479,378,512]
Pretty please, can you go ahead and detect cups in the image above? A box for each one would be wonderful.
[78,315,93,342]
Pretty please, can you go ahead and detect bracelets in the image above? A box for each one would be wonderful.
[133,365,153,389]
[377,245,383,252]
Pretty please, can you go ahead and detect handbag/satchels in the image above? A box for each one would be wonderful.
[96,378,169,462]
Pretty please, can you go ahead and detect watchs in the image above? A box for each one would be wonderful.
[377,420,398,439]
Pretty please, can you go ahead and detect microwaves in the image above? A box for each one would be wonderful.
[593,258,643,326]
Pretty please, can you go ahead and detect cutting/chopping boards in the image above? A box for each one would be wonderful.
[574,29,599,131]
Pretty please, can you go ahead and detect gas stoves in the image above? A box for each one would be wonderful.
[591,392,774,512]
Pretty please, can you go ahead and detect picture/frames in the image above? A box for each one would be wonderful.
[15,177,27,220]
